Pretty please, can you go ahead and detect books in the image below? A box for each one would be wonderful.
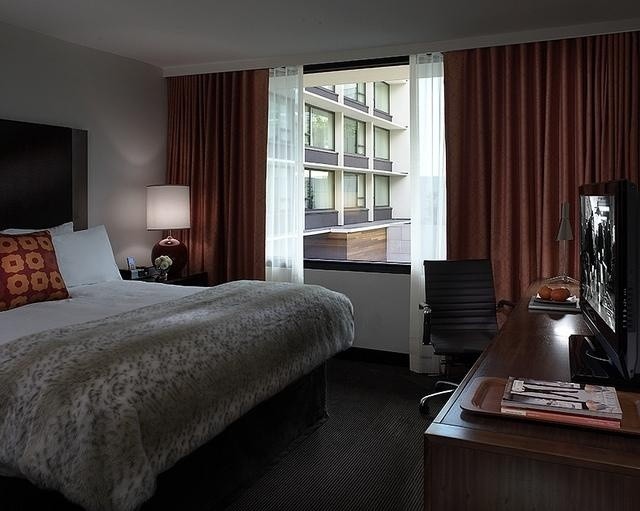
[528,292,582,313]
[500,376,623,430]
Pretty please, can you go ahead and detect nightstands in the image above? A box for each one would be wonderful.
[119,269,208,287]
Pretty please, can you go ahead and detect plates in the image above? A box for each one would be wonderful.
[535,293,577,304]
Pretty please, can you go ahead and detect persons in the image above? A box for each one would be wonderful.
[583,219,613,294]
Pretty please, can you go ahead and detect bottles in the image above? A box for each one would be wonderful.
[136,264,150,277]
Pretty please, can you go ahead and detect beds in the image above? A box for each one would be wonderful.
[1,279,355,511]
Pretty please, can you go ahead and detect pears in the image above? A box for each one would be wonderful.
[539,285,571,301]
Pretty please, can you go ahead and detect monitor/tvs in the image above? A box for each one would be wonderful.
[570,180,640,394]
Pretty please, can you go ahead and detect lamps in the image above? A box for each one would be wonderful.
[543,202,581,285]
[146,184,192,277]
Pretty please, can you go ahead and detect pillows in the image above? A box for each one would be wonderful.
[0,230,73,312]
[51,224,123,281]
[0,222,73,246]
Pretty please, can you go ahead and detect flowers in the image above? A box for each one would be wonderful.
[154,254,173,281]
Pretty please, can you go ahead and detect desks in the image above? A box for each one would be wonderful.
[425,273,640,511]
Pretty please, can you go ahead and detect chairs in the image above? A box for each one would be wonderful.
[419,258,519,415]
